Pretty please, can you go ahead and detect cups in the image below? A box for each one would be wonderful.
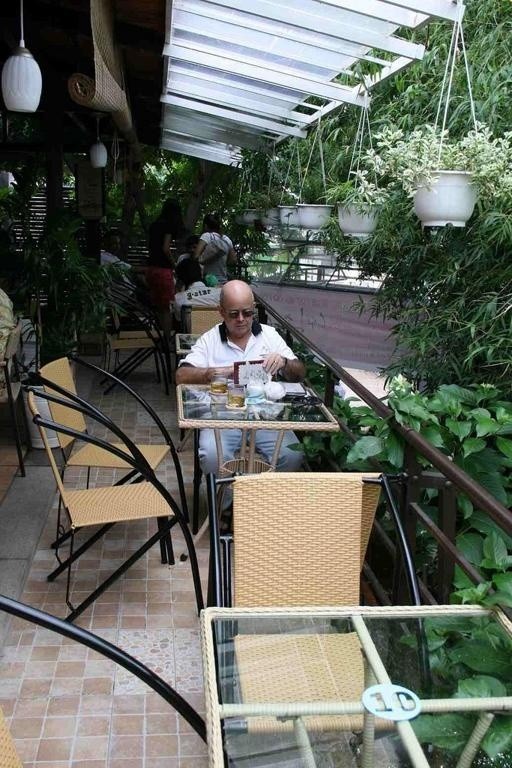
[228,385,245,409]
[211,375,226,392]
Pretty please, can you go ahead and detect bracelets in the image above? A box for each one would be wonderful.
[282,357,288,372]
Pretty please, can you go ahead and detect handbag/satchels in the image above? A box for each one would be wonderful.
[199,240,226,266]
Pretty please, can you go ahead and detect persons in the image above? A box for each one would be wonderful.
[175,279,307,536]
[100,198,234,333]
[0,287,14,399]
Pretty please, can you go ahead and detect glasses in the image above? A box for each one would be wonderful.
[221,305,255,318]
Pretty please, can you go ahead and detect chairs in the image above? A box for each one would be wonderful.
[0,317,40,477]
[225,473,398,734]
[190,307,228,333]
[100,289,172,395]
[27,387,176,622]
[110,280,175,384]
[217,470,382,574]
[23,352,190,524]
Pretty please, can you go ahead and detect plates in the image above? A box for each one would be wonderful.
[246,384,263,399]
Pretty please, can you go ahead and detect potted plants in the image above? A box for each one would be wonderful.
[235,121,512,242]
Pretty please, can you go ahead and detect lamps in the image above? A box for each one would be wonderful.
[91,113,107,168]
[2,0,43,113]
